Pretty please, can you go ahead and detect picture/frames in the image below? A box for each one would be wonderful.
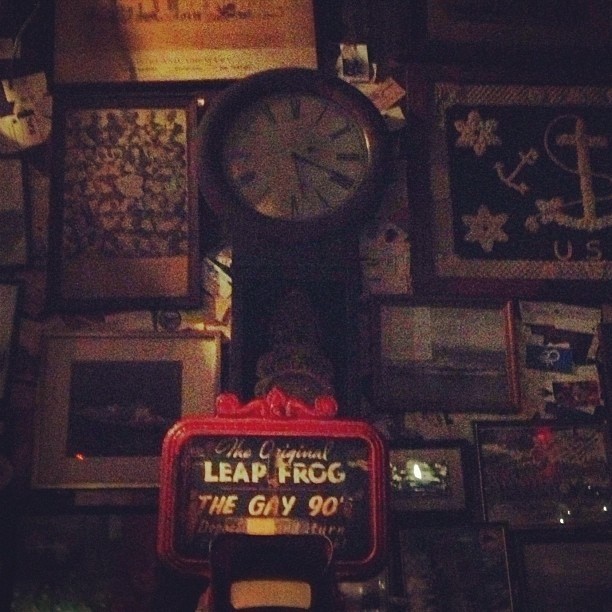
[46,93,206,312]
[2,278,25,406]
[363,292,521,415]
[381,437,476,526]
[0,149,35,276]
[41,1,327,93]
[470,419,612,542]
[372,517,519,611]
[405,62,612,302]
[154,386,386,581]
[30,329,223,491]
[232,277,367,417]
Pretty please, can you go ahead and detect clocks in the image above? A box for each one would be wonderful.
[191,69,394,243]
